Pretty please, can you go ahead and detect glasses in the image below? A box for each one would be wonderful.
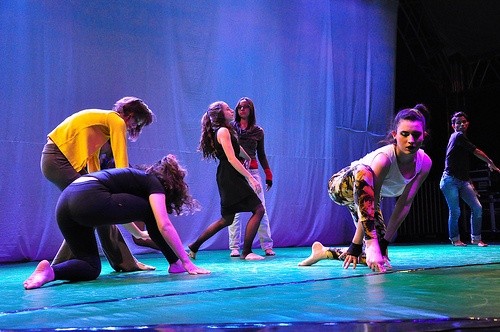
[237,104,250,110]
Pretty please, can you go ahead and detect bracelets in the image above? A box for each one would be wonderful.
[379,237,390,256]
[346,243,362,257]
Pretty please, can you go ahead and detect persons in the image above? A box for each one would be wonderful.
[100,139,162,252]
[439,111,500,246]
[298,103,432,273]
[184,100,266,261]
[228,97,275,257]
[23,153,211,290]
[40,96,155,272]
